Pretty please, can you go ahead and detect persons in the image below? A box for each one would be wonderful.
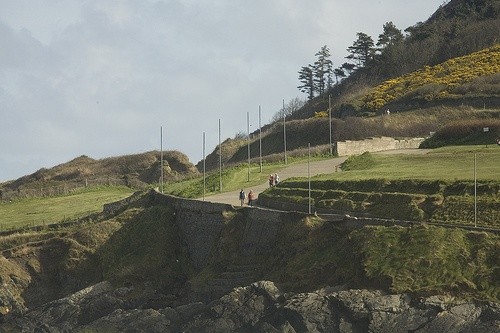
[239,188,246,206]
[268,174,274,187]
[386,109,390,114]
[247,189,255,206]
[273,173,280,186]
